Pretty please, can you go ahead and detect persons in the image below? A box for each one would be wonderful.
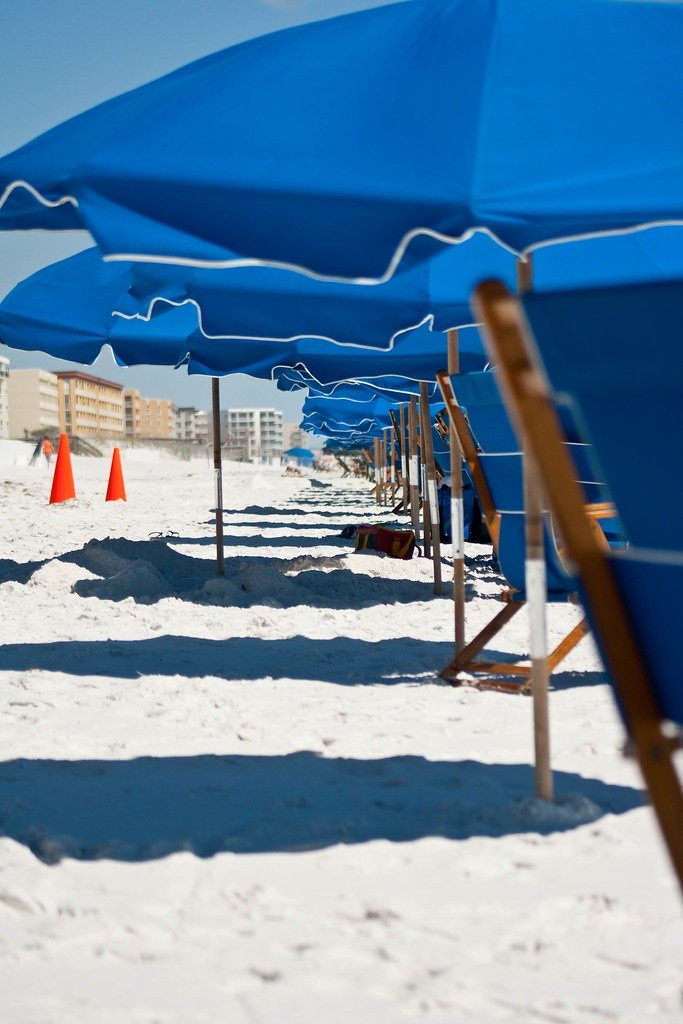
[42,436,56,468]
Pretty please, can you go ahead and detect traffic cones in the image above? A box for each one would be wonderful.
[104,447,127,502]
[46,431,78,505]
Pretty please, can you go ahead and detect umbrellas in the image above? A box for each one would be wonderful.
[0,0,683,799]
[284,448,313,466]
[1,247,496,596]
[107,221,683,658]
